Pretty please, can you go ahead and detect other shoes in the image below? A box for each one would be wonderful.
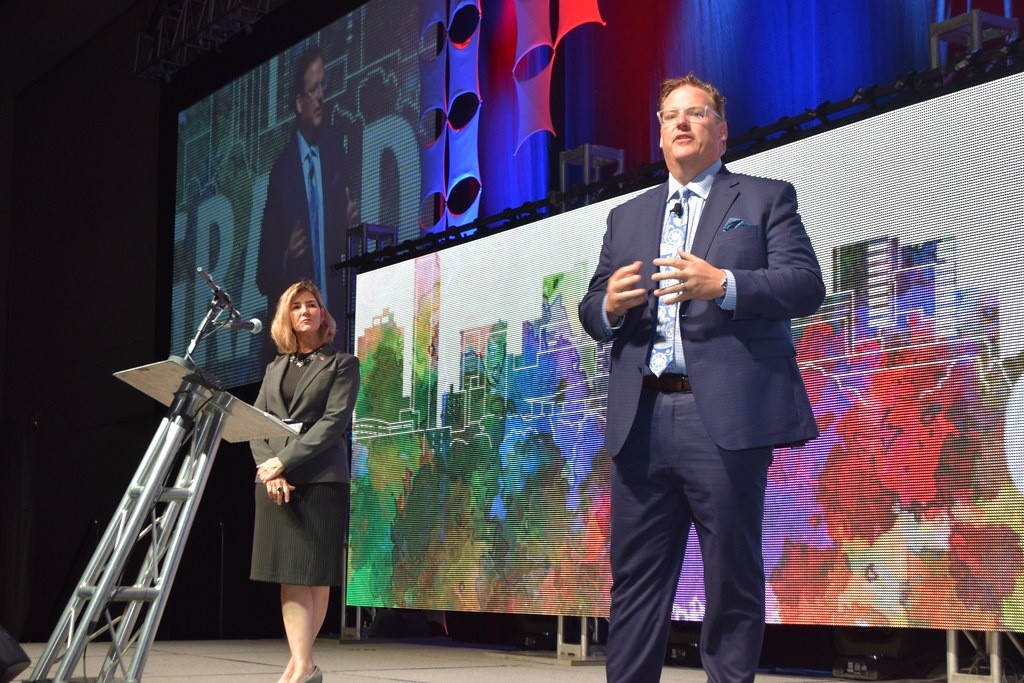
[303,665,323,683]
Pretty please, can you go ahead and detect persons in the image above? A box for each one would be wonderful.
[255,45,361,360]
[578,73,826,683]
[249,281,361,683]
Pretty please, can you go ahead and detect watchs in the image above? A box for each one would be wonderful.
[721,274,727,299]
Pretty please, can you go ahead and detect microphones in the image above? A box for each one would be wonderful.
[670,203,682,217]
[215,317,263,334]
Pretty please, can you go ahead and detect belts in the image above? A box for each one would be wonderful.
[641,372,693,393]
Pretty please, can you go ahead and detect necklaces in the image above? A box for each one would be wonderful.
[289,346,323,368]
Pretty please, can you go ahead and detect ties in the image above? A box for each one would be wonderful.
[647,186,692,377]
[303,151,323,294]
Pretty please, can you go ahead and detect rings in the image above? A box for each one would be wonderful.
[277,486,282,491]
[681,283,685,291]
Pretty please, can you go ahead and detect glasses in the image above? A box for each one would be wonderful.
[656,106,721,127]
[299,80,325,99]
